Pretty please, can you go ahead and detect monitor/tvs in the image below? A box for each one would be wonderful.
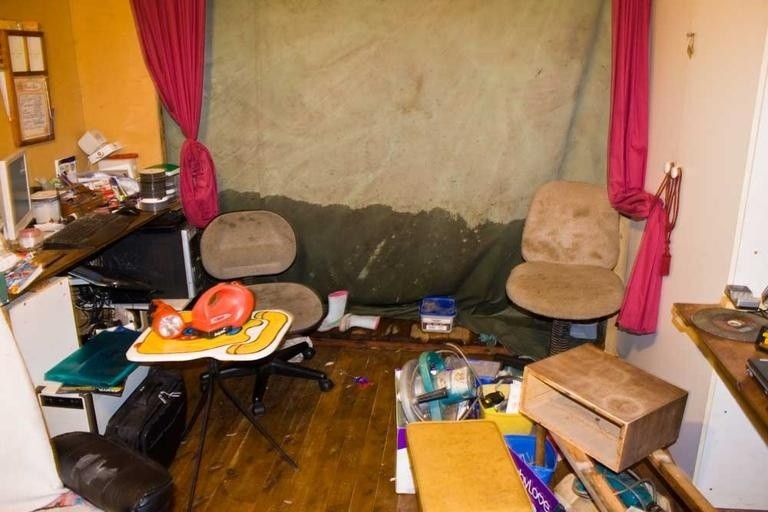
[0,150,33,243]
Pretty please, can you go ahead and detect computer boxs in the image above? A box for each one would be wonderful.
[99,222,206,299]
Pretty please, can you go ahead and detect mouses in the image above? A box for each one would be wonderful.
[118,205,140,217]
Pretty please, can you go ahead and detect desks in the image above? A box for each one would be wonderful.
[0,192,185,452]
[123,309,299,512]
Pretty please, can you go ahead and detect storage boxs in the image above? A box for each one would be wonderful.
[519,342,689,473]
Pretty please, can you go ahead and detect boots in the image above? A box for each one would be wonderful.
[317,290,381,332]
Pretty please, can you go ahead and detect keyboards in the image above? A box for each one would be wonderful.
[41,209,117,250]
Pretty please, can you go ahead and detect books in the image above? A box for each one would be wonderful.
[4,262,44,295]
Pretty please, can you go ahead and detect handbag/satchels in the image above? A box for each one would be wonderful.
[104,366,187,470]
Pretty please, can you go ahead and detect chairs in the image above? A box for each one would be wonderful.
[505,178,625,359]
[198,207,336,418]
